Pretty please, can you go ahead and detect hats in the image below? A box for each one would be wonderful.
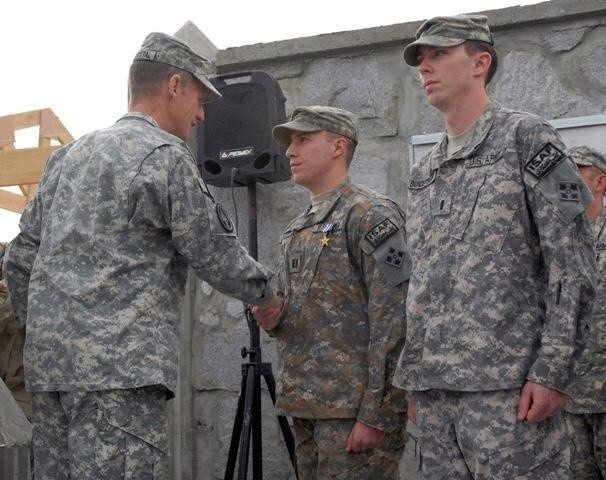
[566,143,606,174]
[403,15,494,67]
[132,33,223,104]
[272,106,359,146]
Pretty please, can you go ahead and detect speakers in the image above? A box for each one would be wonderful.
[195,69,292,189]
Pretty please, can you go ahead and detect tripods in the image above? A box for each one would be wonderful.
[223,174,299,480]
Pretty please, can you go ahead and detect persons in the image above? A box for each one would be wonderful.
[563,145,606,480]
[1,28,284,480]
[394,14,598,480]
[247,104,412,480]
